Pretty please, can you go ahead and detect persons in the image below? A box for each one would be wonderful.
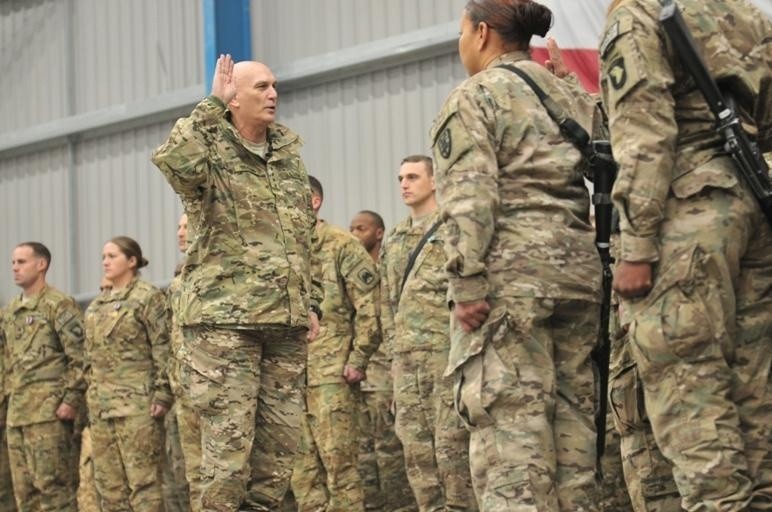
[151,55,325,512]
[163,209,191,512]
[607,278,682,511]
[77,275,112,512]
[377,155,478,510]
[351,212,418,511]
[597,1,772,512]
[288,177,379,512]
[429,1,612,511]
[81,236,172,512]
[0,242,86,512]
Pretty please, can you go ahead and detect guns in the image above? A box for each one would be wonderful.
[661,0,772,205]
[569,141,616,484]
